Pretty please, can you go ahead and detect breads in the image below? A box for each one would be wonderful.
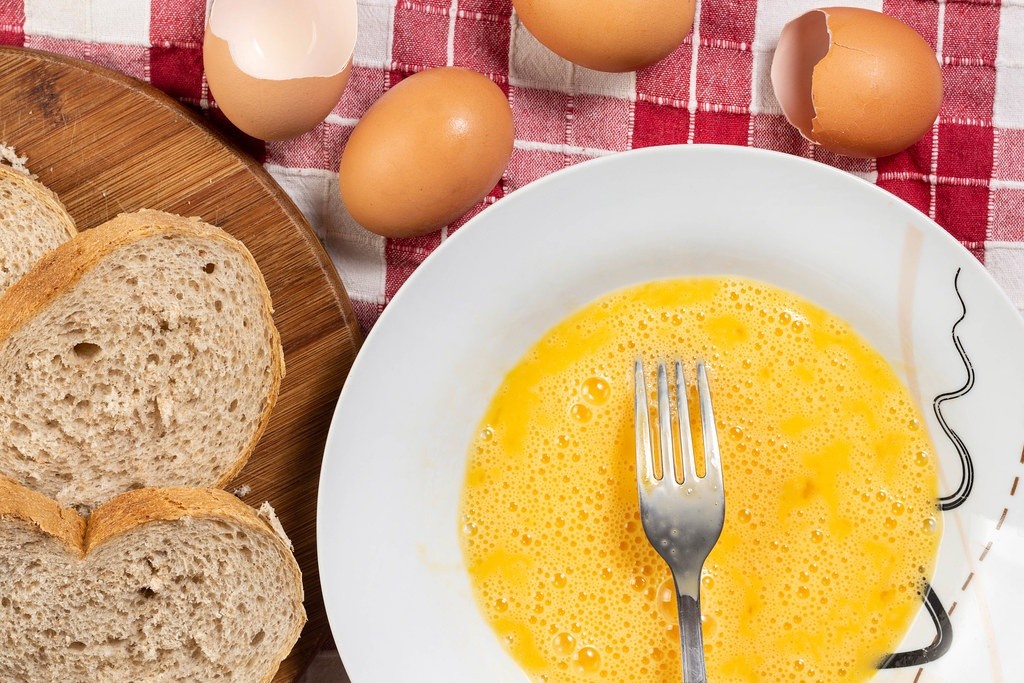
[0,157,308,683]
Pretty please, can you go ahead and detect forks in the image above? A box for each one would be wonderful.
[634,361,725,683]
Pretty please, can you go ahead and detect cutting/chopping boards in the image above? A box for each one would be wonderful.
[2,45,362,683]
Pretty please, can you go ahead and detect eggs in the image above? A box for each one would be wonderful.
[340,65,515,238]
[511,0,697,73]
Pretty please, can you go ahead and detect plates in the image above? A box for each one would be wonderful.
[316,144,1024,683]
